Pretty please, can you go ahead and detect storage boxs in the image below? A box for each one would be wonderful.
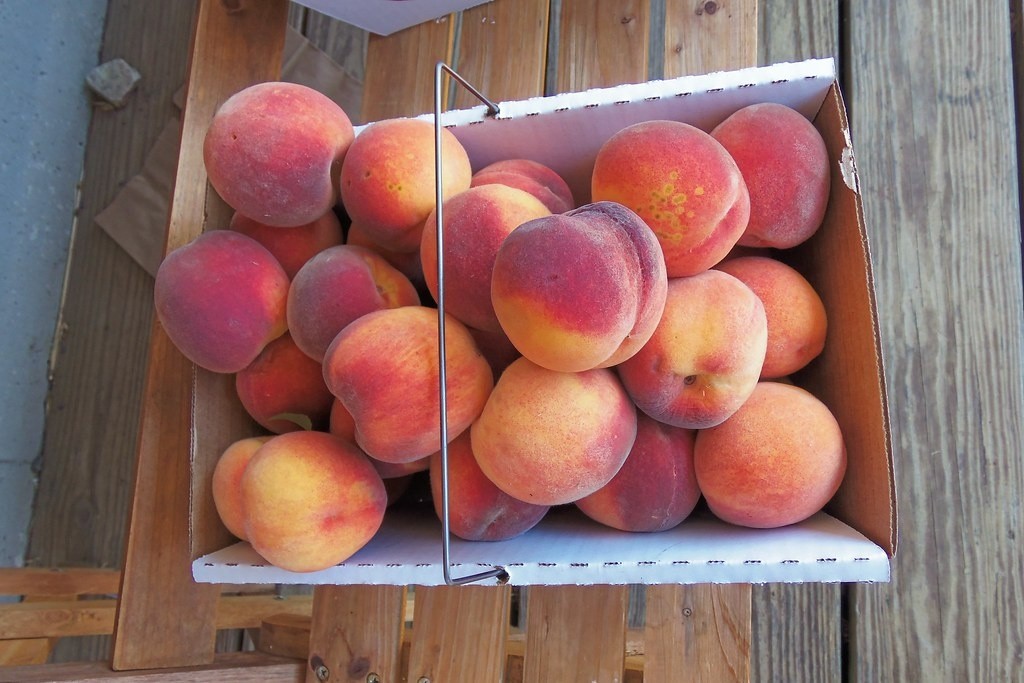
[189,55,900,587]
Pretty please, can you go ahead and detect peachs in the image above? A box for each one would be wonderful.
[155,82,848,574]
